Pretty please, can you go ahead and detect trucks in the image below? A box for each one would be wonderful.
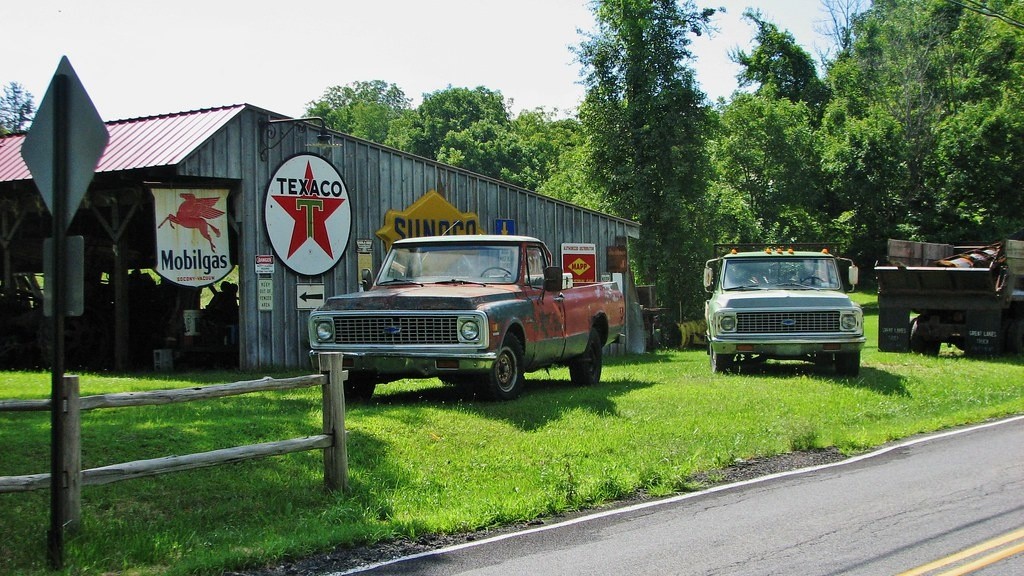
[874,239,1024,358]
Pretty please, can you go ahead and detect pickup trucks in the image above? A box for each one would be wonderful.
[307,234,627,402]
[702,243,867,377]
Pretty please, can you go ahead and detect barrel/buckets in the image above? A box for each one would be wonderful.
[183,309,201,336]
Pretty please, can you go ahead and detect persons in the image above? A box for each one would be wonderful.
[203,279,240,369]
